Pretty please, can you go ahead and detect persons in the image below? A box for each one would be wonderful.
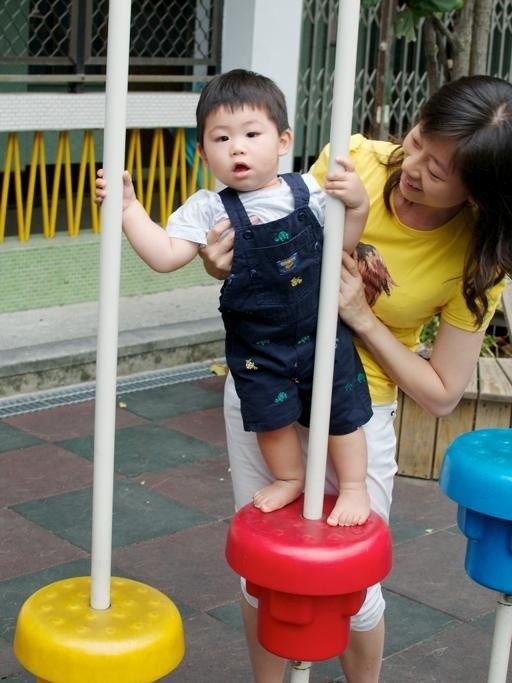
[93,70,370,527]
[199,75,512,683]
